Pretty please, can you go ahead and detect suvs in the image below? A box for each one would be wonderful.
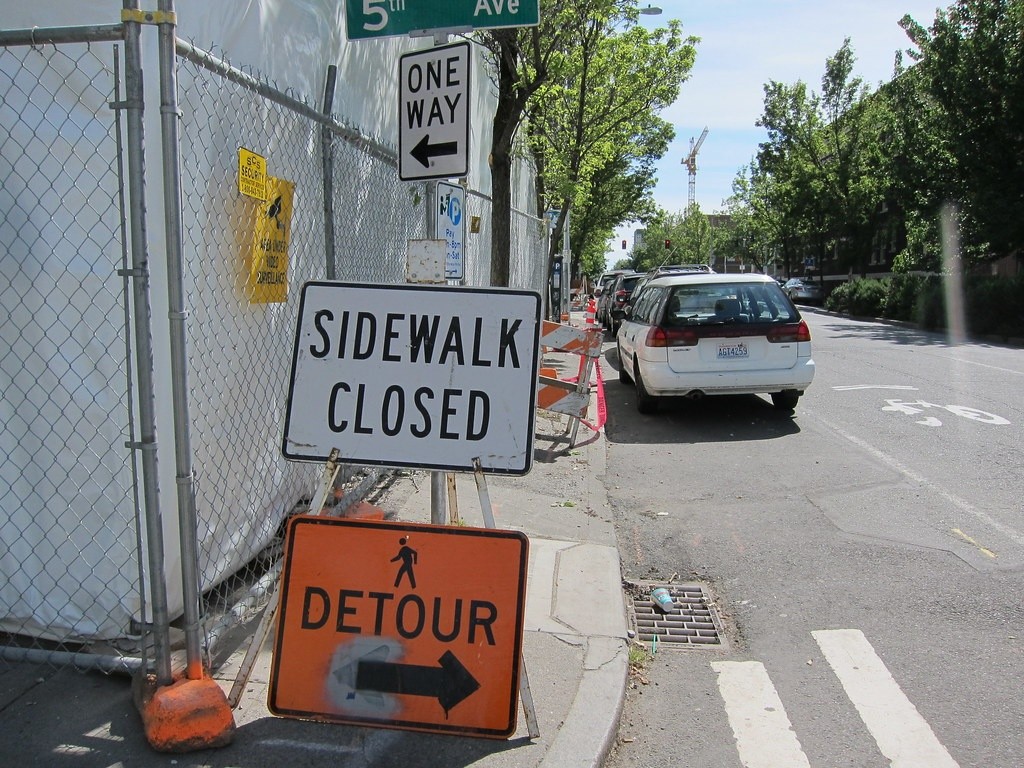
[622,264,718,319]
[605,273,647,336]
[594,270,636,319]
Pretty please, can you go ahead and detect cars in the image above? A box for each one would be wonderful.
[596,280,615,324]
[781,277,822,303]
[616,271,816,409]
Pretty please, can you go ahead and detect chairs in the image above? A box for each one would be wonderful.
[706,298,748,324]
[670,296,682,314]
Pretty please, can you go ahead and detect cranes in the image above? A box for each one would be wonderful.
[680,125,709,215]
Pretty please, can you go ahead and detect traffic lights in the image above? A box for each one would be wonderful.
[622,241,626,249]
[665,240,670,249]
